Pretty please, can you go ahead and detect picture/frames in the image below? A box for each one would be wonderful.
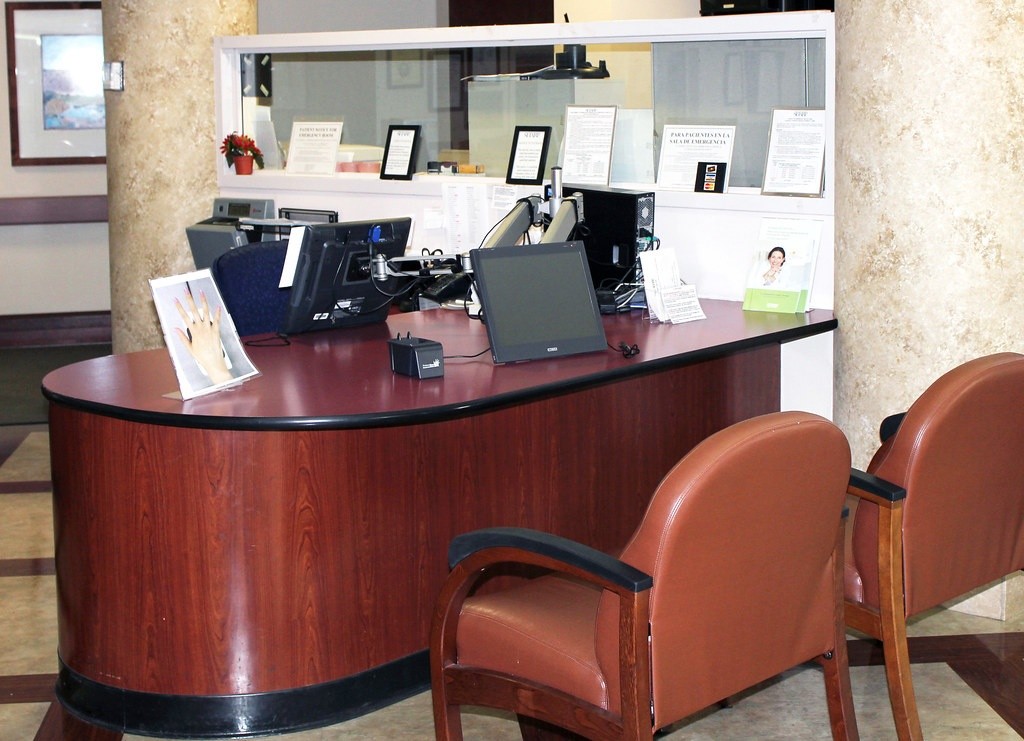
[378,125,421,181]
[4,1,107,167]
[506,126,552,185]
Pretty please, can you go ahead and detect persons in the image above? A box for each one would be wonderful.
[762,247,786,286]
[171,286,236,386]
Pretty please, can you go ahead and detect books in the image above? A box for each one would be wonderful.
[742,218,823,314]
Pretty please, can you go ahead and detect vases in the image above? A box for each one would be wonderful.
[235,156,254,174]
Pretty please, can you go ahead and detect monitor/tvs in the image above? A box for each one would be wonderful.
[281,218,412,335]
[470,241,608,363]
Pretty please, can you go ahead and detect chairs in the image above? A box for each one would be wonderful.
[212,239,289,335]
[429,410,860,741]
[846,352,1024,741]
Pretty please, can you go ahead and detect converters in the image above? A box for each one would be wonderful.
[597,290,616,314]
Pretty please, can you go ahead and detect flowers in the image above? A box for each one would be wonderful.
[220,129,265,169]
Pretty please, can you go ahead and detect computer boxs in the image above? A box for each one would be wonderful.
[543,183,656,302]
